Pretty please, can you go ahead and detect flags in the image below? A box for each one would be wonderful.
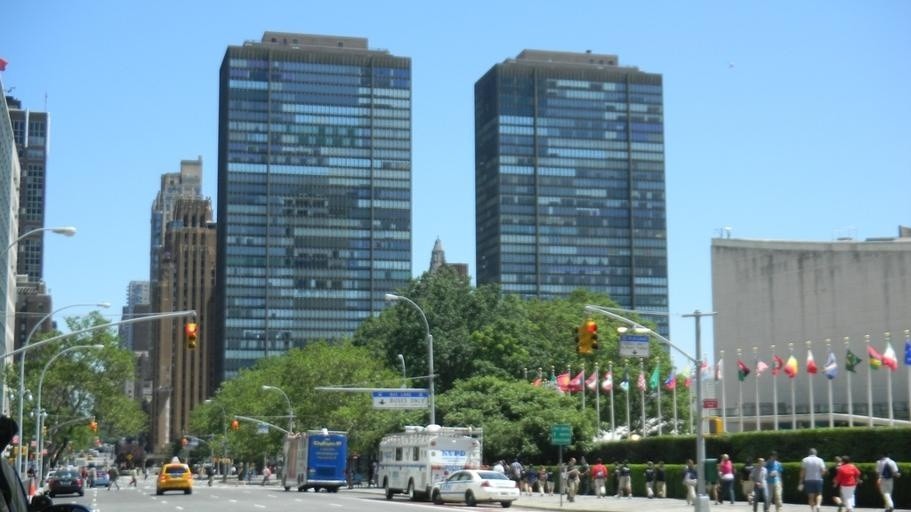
[821,347,838,379]
[755,357,769,376]
[532,357,724,395]
[770,355,783,376]
[735,358,750,381]
[805,350,817,374]
[902,340,911,365]
[881,341,898,372]
[866,346,882,369]
[783,354,799,378]
[845,349,862,372]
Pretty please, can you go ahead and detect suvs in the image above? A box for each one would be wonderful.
[156,456,194,494]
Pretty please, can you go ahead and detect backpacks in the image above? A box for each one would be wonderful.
[883,463,892,479]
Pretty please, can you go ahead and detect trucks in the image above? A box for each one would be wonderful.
[281,430,349,493]
[377,424,485,501]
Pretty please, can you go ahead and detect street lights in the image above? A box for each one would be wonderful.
[34,343,107,478]
[261,385,293,434]
[0,226,76,264]
[397,352,408,389]
[384,292,436,424]
[18,303,113,481]
[203,399,228,457]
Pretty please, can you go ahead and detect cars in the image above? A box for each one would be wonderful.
[430,468,522,507]
[192,458,237,474]
[43,443,115,497]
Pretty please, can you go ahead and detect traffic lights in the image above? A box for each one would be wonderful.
[181,438,189,446]
[232,420,240,430]
[587,319,599,356]
[88,422,98,432]
[186,322,198,351]
[572,325,589,355]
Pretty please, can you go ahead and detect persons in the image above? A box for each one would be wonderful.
[705,447,861,512]
[875,449,902,511]
[345,458,378,489]
[493,457,698,506]
[28,461,272,491]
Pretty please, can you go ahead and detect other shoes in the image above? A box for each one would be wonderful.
[714,498,894,512]
[526,489,670,503]
